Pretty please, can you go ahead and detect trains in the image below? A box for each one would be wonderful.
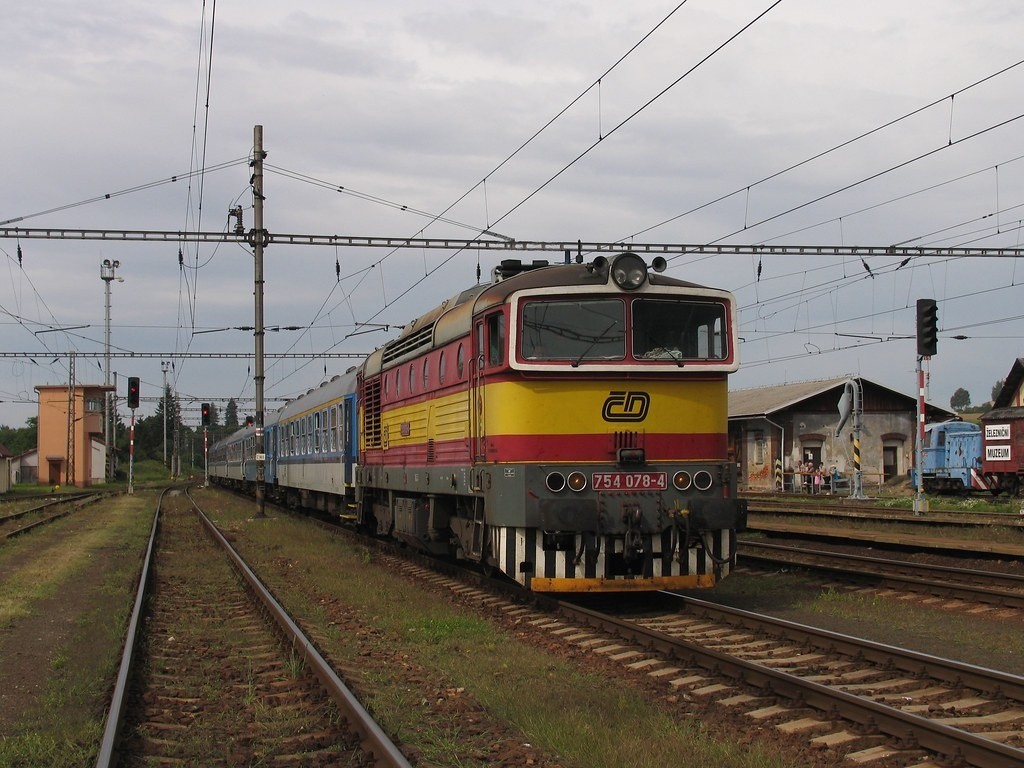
[207,252,749,612]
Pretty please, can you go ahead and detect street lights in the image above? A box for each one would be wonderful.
[160,359,175,468]
[100,259,125,481]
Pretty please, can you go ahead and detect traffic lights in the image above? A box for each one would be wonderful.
[917,298,938,356]
[202,403,211,427]
[246,416,254,427]
[128,377,139,408]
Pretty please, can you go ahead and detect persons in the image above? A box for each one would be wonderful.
[532,344,547,357]
[798,460,838,493]
